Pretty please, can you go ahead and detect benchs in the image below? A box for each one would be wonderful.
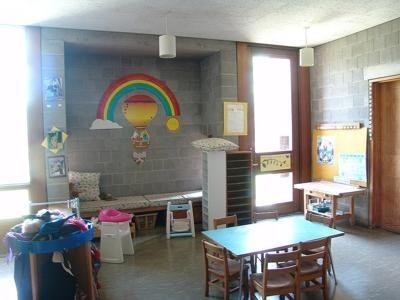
[72,189,202,218]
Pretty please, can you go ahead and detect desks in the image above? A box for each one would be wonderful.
[201,216,344,300]
[294,181,368,228]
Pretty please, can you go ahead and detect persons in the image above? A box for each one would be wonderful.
[49,135,61,149]
[51,160,64,174]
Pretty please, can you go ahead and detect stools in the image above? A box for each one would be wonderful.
[98,221,135,263]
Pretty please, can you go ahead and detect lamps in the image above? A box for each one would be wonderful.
[159,15,177,58]
[299,26,314,66]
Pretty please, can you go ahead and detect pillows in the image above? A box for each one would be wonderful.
[190,138,239,152]
[68,171,101,201]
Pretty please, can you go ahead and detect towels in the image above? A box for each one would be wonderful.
[98,209,134,222]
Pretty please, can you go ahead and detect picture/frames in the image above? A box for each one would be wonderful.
[224,102,248,136]
[47,155,66,178]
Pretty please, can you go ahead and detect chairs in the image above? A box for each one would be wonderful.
[250,248,302,300]
[278,236,331,300]
[253,209,288,272]
[202,240,249,300]
[213,214,237,229]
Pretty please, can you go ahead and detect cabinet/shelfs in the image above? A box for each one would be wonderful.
[226,148,253,227]
[202,151,226,230]
